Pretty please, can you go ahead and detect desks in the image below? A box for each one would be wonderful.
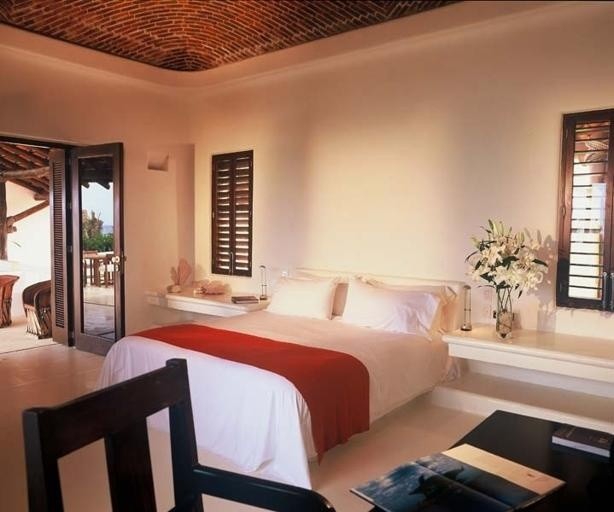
[367,406,613,512]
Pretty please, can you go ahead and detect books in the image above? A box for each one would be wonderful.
[231,296,258,304]
[347,442,567,511]
[551,424,613,458]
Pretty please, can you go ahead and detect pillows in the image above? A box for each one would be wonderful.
[263,273,454,343]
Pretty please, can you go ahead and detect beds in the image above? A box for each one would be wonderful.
[103,266,468,481]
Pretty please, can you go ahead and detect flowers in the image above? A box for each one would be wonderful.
[461,215,552,334]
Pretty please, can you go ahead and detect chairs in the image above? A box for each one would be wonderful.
[19,355,334,511]
[20,278,52,339]
[0,274,19,328]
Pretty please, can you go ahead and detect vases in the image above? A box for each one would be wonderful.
[491,297,517,340]
